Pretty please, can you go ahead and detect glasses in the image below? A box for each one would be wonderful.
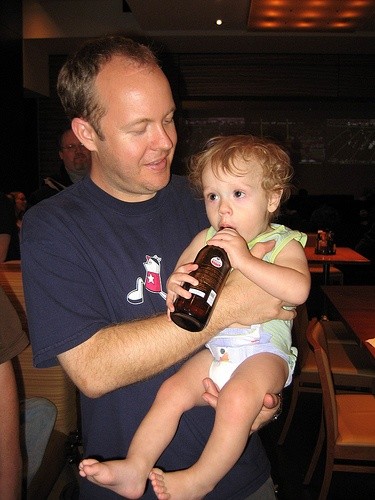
[61,143,86,152]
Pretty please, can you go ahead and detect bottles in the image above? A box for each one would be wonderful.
[171,226,235,332]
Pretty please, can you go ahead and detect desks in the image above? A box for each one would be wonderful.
[303,246,371,284]
[321,286,375,359]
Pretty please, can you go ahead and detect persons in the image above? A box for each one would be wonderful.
[0,287,30,500]
[0,127,92,263]
[20,36,297,500]
[79,135,312,500]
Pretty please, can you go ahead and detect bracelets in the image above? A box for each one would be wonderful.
[274,393,283,420]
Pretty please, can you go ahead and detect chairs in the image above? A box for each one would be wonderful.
[306,316,375,500]
[0,262,79,500]
[279,282,374,443]
[303,232,343,285]
